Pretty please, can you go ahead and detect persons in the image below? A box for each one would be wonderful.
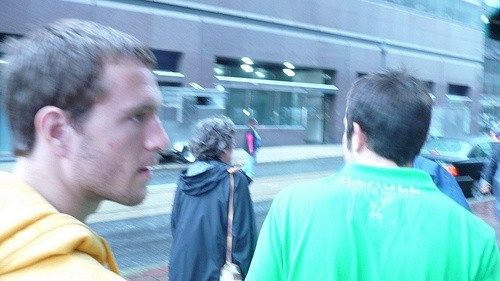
[242,116,262,183]
[412,154,471,212]
[0,18,170,281]
[166,115,258,281]
[242,70,500,281]
[480,126,500,222]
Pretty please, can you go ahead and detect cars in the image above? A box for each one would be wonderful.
[421,134,499,198]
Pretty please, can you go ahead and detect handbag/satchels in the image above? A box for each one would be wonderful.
[219,173,242,281]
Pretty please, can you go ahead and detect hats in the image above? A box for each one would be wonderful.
[248,117,258,125]
